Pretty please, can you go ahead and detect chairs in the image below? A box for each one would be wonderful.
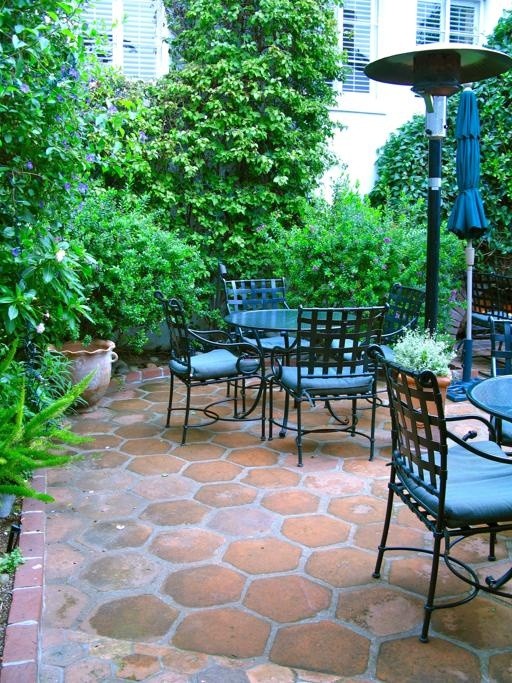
[153,291,267,445]
[325,282,425,410]
[267,303,387,468]
[465,271,512,364]
[373,358,510,644]
[221,275,311,415]
[483,315,512,374]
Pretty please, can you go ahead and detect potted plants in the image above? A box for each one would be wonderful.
[397,371,451,429]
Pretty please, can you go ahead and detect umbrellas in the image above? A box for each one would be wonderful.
[448,83,490,382]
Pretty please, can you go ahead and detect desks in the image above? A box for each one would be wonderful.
[467,374,509,444]
[223,308,364,425]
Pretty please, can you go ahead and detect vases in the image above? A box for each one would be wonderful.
[44,339,118,413]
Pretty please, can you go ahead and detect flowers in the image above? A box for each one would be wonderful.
[389,323,454,379]
